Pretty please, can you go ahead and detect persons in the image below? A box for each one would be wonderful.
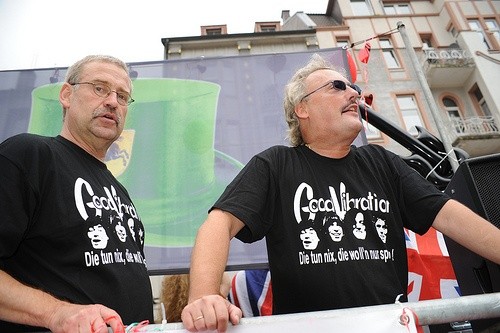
[180,54,500,332]
[0,54,154,333]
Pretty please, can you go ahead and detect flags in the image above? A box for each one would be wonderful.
[227,225,462,321]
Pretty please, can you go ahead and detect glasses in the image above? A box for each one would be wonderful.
[70,82,135,106]
[300,79,362,103]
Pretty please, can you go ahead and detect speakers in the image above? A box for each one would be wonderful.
[441,151,500,333]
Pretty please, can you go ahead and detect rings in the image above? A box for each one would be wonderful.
[193,315,204,322]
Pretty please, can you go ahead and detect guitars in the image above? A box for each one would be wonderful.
[353,86,471,192]
